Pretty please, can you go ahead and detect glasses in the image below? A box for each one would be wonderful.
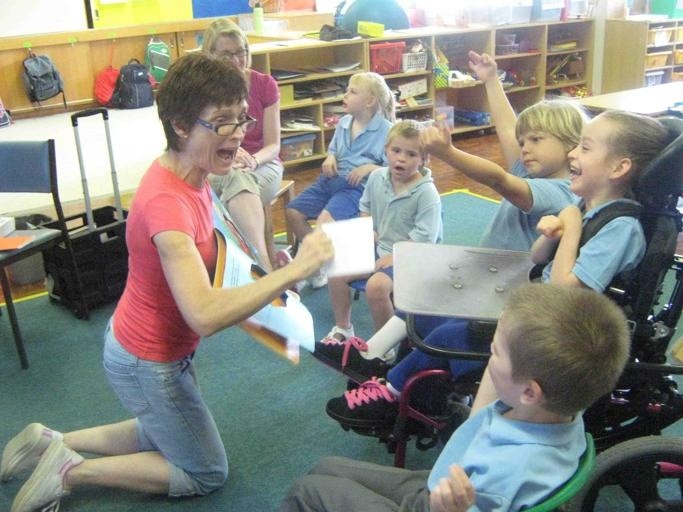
[213,48,248,60]
[196,114,257,136]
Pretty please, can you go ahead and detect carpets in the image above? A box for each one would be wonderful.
[0,189,683,511]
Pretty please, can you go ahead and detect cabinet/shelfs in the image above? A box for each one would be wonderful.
[184,15,683,166]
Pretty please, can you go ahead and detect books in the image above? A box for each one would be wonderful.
[272,62,363,80]
[210,189,314,365]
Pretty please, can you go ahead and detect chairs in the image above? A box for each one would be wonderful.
[0,140,90,369]
[521,433,596,512]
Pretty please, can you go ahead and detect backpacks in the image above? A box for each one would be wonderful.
[94,35,172,109]
[0,97,14,129]
[22,48,65,103]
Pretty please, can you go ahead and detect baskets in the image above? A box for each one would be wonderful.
[369,42,428,75]
[645,26,683,87]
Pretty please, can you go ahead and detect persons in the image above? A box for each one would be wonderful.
[421,50,587,255]
[284,71,443,360]
[1,51,334,511]
[203,17,285,273]
[309,108,670,427]
[278,281,630,511]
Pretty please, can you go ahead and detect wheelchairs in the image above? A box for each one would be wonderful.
[334,113,683,512]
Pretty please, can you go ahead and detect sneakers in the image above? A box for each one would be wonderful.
[1,423,84,512]
[307,324,399,427]
[275,249,328,293]
